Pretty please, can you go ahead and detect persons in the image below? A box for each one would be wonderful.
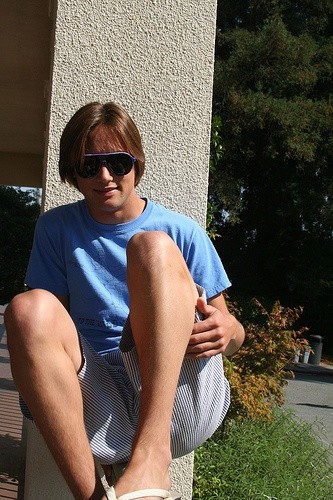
[4,99,245,500]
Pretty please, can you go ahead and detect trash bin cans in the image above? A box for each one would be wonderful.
[309,335,324,364]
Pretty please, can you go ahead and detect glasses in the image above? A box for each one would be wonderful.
[75,152,138,178]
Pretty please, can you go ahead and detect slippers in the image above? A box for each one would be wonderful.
[95,461,118,493]
[106,484,182,500]
